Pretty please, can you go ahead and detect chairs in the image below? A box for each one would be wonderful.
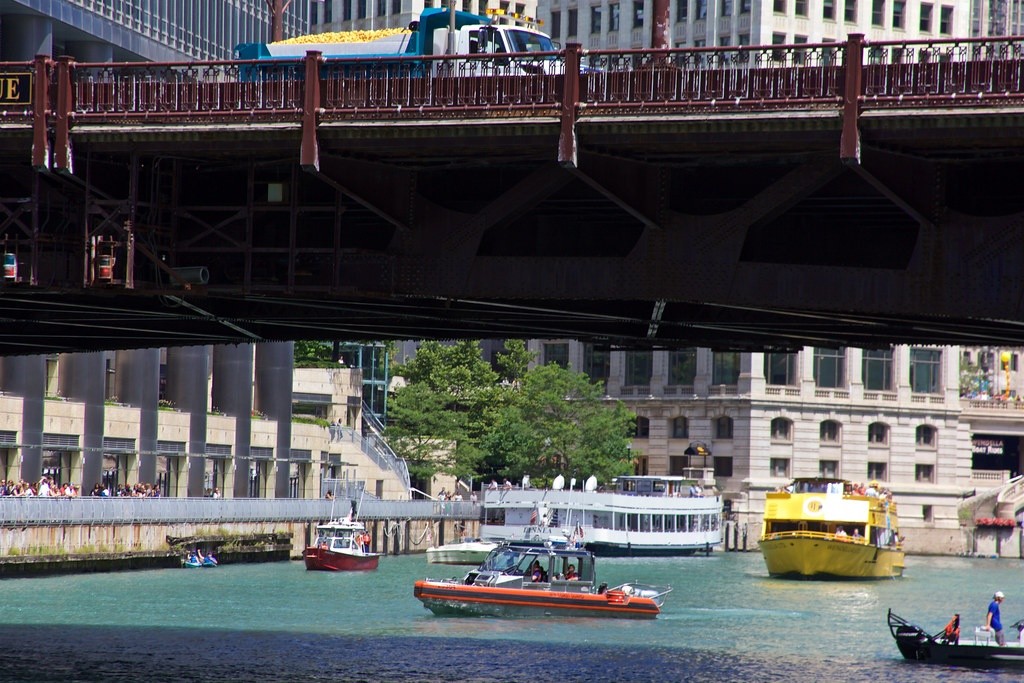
[469,39,506,65]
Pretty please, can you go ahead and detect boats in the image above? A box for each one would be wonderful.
[757,477,908,582]
[413,507,675,620]
[182,562,218,569]
[886,609,1024,671]
[424,530,499,566]
[478,476,724,558]
[300,476,386,574]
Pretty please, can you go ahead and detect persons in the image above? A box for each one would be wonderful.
[438,478,513,543]
[205,488,222,497]
[689,483,704,497]
[0,475,80,496]
[770,483,898,546]
[470,40,528,65]
[941,613,960,645]
[90,482,161,497]
[325,490,335,501]
[960,390,1024,400]
[985,591,1024,647]
[188,549,217,563]
[506,559,578,584]
[538,514,549,527]
[316,530,371,555]
[329,418,344,442]
[502,376,522,384]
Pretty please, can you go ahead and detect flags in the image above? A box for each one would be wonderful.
[530,504,537,523]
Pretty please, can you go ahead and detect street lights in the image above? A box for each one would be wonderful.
[625,443,635,465]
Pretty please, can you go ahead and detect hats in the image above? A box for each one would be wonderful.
[993,591,1005,599]
[190,551,196,556]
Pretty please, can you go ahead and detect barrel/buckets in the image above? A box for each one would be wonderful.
[606,591,625,604]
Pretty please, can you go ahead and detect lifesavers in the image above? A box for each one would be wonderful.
[803,496,825,517]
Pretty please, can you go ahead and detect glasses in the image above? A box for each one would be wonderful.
[2,482,6,483]
